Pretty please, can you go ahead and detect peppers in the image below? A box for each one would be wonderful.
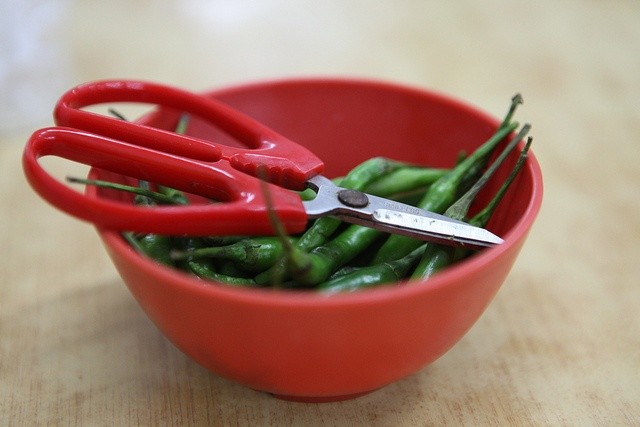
[67,92,536,291]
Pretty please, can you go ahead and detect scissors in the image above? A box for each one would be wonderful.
[23,80,504,248]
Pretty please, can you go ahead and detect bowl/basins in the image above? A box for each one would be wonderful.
[82,74,545,403]
